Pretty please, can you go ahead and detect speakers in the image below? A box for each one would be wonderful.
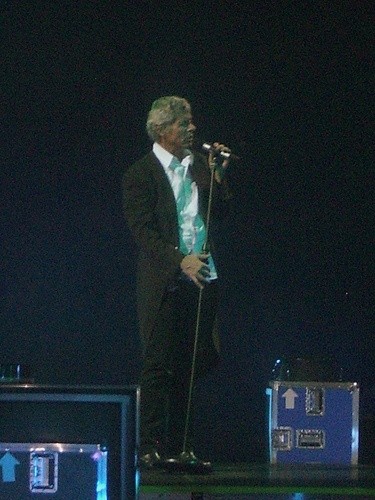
[0,383,139,500]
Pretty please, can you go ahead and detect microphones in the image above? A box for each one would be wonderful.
[198,141,241,162]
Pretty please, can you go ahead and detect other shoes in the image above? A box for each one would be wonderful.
[138,447,166,472]
[167,450,213,474]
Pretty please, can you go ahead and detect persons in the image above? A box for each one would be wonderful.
[122,96,233,473]
